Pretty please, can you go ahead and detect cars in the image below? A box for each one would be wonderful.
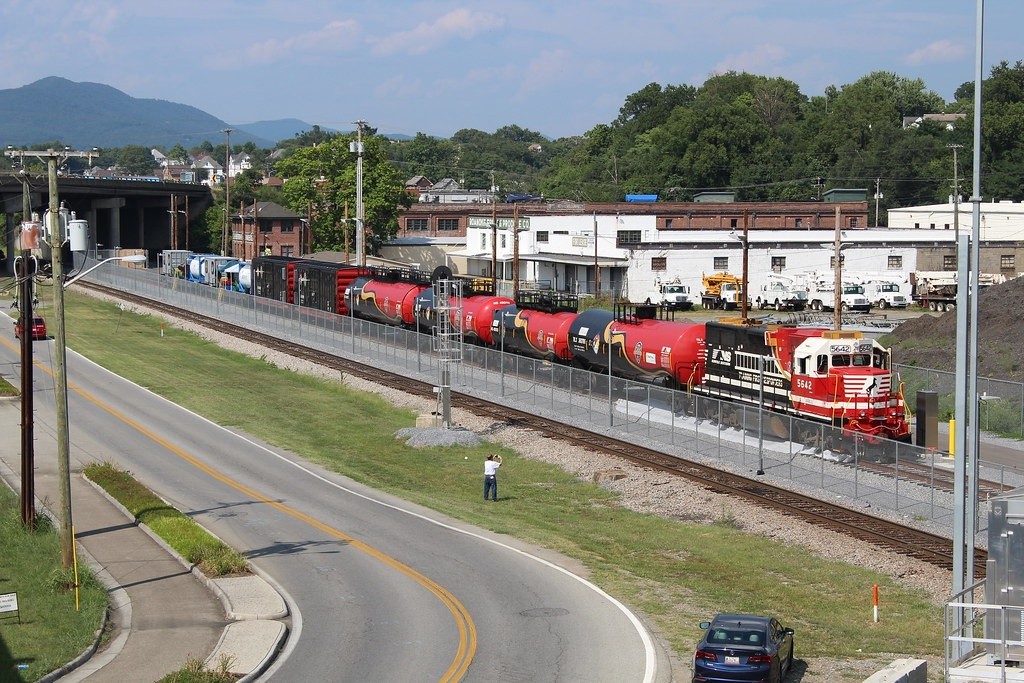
[691,611,796,682]
[13,316,46,341]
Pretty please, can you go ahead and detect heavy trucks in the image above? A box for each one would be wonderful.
[701,282,743,311]
[911,270,1006,312]
[755,282,907,312]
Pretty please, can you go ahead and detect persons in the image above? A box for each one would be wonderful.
[483,453,503,502]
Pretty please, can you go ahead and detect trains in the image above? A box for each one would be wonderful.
[160,246,916,459]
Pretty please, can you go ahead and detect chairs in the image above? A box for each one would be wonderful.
[714,632,727,639]
[749,635,759,642]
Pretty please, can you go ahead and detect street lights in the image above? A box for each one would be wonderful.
[53,253,149,575]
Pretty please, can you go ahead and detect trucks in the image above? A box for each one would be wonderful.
[645,284,693,311]
